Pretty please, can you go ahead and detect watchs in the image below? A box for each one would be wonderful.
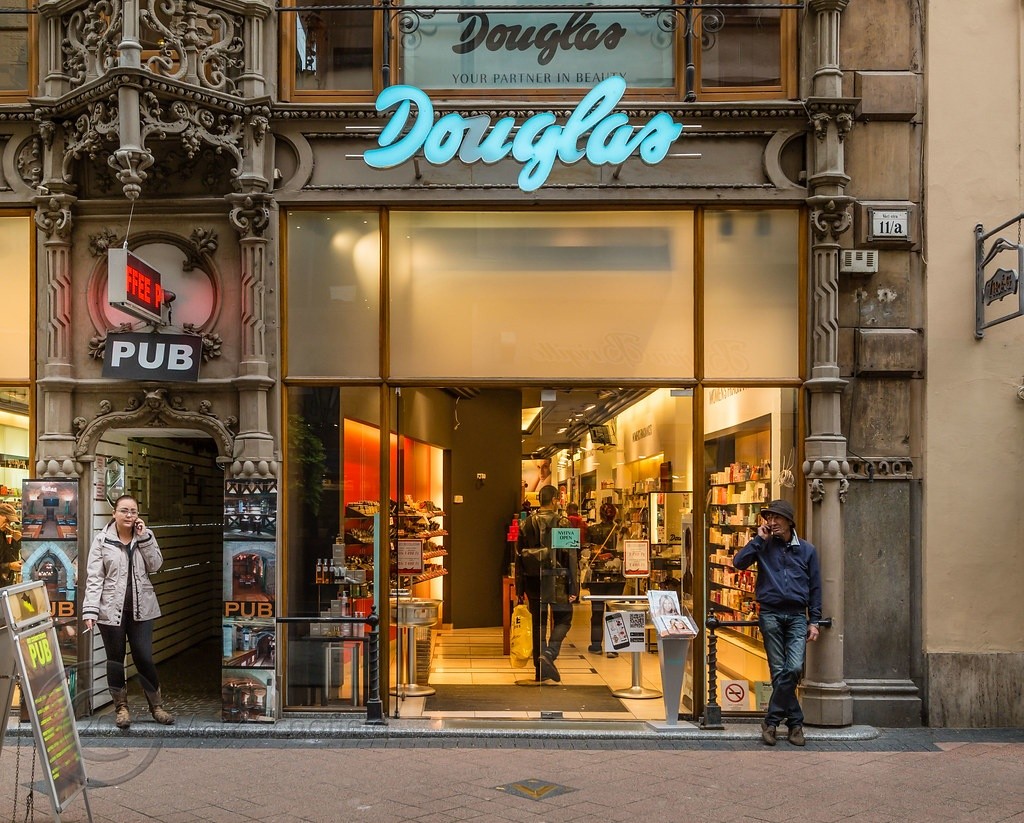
[810,622,820,628]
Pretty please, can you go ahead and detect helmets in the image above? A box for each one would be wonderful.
[600,503,616,517]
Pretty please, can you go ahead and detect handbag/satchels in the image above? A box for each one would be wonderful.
[509,596,533,668]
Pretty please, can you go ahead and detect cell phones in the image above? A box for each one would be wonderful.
[765,525,772,534]
[134,522,143,530]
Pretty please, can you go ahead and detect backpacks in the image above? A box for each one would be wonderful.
[520,514,563,605]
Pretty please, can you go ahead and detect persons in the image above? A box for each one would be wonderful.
[732,500,823,744]
[528,458,551,492]
[82,494,175,728]
[514,485,578,683]
[0,503,22,588]
[683,527,692,598]
[655,594,679,617]
[583,503,626,659]
[565,502,588,603]
[668,618,694,634]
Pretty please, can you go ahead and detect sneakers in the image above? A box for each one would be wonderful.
[761,722,777,745]
[539,651,561,682]
[536,665,551,682]
[607,652,619,657]
[789,725,805,746]
[589,643,602,654]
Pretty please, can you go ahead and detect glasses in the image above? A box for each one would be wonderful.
[114,509,140,516]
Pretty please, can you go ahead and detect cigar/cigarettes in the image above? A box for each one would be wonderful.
[82,627,90,634]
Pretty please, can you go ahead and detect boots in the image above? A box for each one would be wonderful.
[108,683,131,728]
[144,681,175,724]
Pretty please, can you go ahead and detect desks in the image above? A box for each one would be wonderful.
[390,596,441,699]
[502,572,530,656]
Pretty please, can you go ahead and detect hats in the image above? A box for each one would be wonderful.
[761,499,796,529]
[0,503,20,525]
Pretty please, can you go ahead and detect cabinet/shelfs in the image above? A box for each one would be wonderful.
[524,474,777,665]
[343,498,449,589]
